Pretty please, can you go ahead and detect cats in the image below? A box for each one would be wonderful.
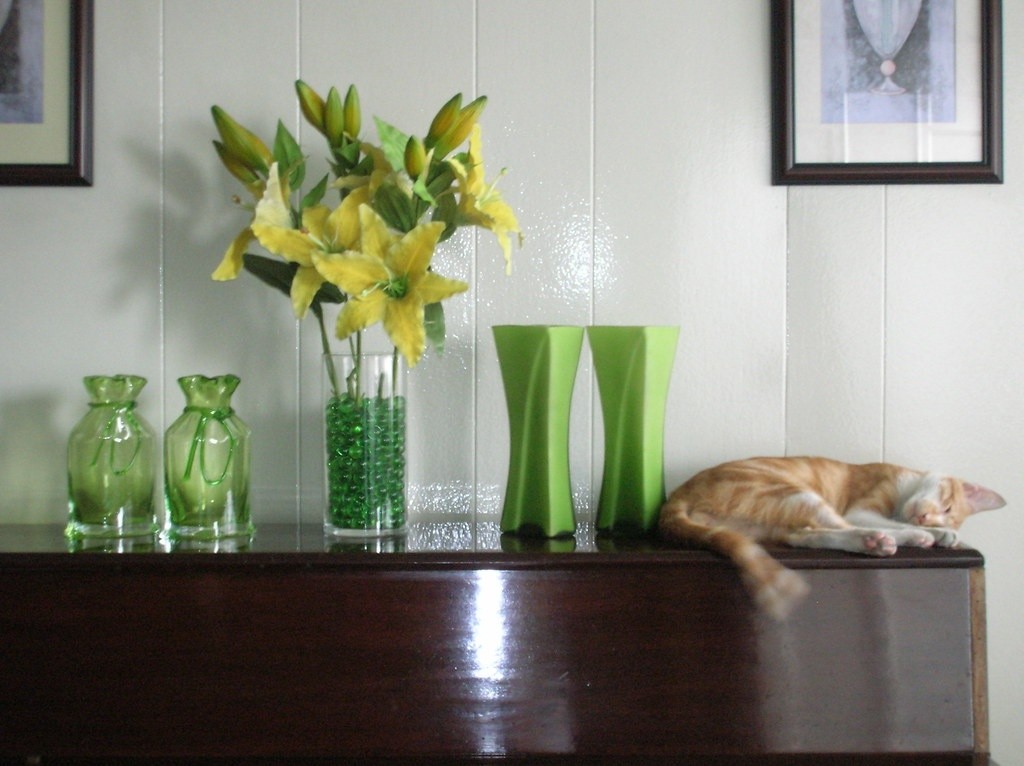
[657,456,1007,622]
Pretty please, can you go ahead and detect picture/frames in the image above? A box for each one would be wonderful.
[770,0,1004,186]
[0,0,94,188]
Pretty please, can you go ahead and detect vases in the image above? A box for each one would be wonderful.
[585,326,679,539]
[63,374,160,538]
[321,352,409,545]
[163,374,254,542]
[492,325,585,538]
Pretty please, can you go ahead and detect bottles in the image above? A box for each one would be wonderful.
[64,375,157,539]
[164,375,258,539]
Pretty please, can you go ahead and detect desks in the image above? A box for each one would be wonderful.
[1,523,988,766]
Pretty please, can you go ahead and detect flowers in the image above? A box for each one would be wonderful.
[210,78,526,398]
